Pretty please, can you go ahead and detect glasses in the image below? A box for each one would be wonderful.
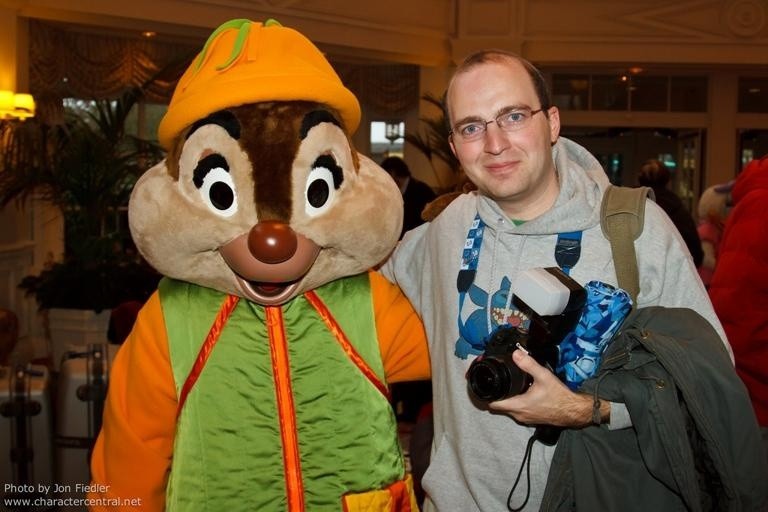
[448,106,546,140]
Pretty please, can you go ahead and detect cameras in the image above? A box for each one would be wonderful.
[466,266,587,409]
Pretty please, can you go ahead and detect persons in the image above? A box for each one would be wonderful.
[638,159,708,268]
[367,51,739,510]
[377,154,437,237]
[76,16,436,510]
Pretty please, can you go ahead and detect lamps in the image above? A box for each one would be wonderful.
[0,88,37,123]
